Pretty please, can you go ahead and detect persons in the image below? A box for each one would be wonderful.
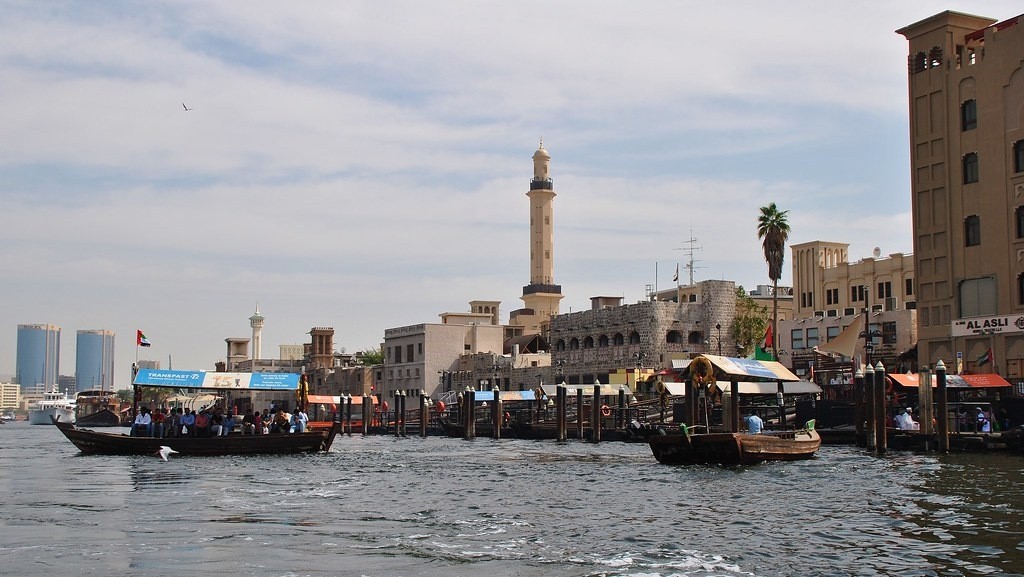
[901,407,920,430]
[742,410,764,432]
[129,405,309,436]
[831,373,854,397]
[961,407,1006,433]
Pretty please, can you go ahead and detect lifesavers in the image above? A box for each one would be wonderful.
[602,405,610,417]
[296,382,309,401]
[656,381,666,394]
[690,356,713,384]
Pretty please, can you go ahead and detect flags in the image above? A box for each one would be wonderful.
[137,330,151,347]
[976,347,995,367]
[765,326,773,346]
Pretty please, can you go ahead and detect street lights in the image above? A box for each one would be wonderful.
[704,323,723,356]
[491,361,503,386]
[555,359,567,383]
[858,329,882,364]
[632,351,647,371]
[437,368,450,393]
[766,349,789,362]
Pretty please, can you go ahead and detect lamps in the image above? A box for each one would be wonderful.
[833,316,841,321]
[798,320,805,324]
[854,314,861,319]
[873,312,882,317]
[816,318,823,324]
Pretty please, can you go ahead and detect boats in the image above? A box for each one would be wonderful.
[75,375,121,427]
[29,384,76,425]
[645,417,828,468]
[885,372,1012,446]
[0,410,16,420]
[48,361,343,457]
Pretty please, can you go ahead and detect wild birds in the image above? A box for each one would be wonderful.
[183,103,195,112]
[154,445,180,462]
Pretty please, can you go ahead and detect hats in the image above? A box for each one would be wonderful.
[198,409,204,414]
[293,409,300,413]
[906,407,912,413]
[976,406,982,410]
